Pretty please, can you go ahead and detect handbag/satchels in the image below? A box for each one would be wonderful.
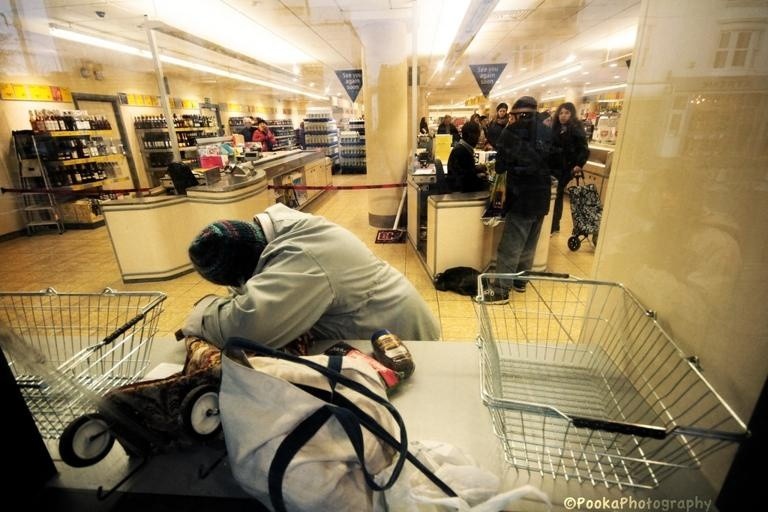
[433,266,489,298]
[217,335,459,512]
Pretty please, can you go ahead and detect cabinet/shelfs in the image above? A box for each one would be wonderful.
[134,127,218,187]
[14,129,131,230]
[229,125,297,152]
[302,118,340,175]
[339,121,367,174]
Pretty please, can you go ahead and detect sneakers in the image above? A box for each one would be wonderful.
[512,282,527,293]
[473,287,511,306]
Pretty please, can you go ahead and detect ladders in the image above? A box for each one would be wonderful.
[13,130,63,237]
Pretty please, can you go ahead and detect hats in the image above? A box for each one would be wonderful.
[508,96,538,114]
[187,218,269,289]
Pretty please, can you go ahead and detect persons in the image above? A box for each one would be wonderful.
[420,97,590,236]
[180,202,443,352]
[472,94,563,306]
[237,115,277,152]
[297,121,305,149]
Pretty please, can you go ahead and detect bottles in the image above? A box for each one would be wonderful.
[28,109,117,187]
[302,105,367,168]
[133,113,217,148]
[371,329,416,379]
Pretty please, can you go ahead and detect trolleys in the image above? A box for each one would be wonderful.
[58,297,317,503]
[567,170,605,252]
